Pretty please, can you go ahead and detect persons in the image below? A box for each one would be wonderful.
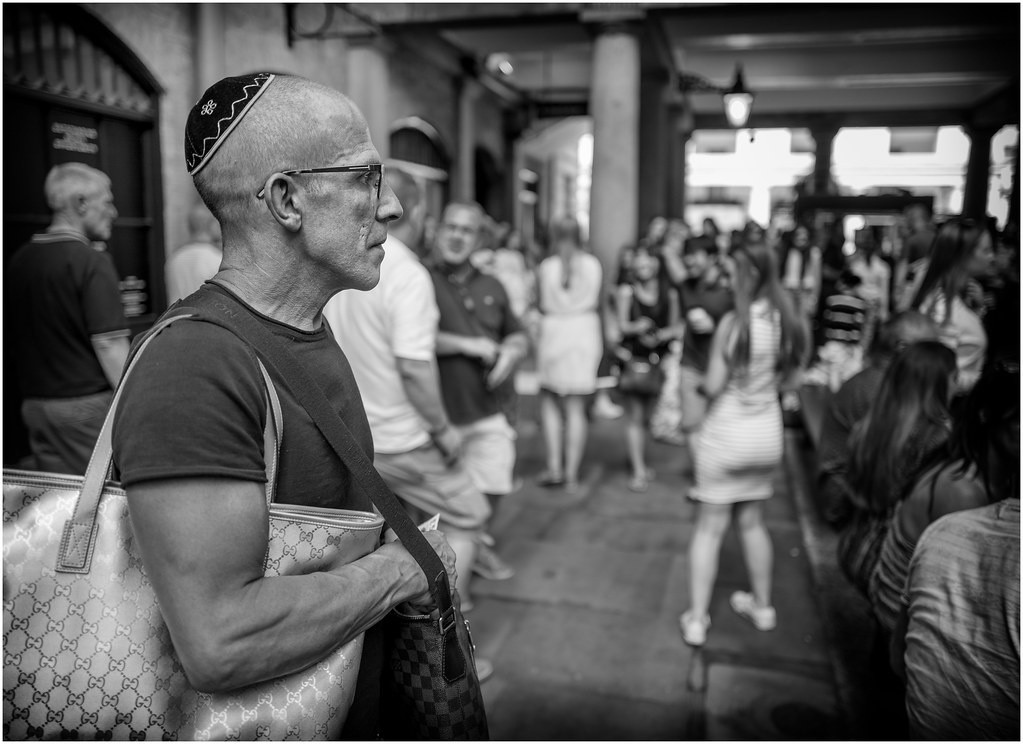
[165,197,221,302]
[102,69,458,742]
[321,165,1021,742]
[3,162,132,482]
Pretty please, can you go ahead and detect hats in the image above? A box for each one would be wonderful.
[185,72,275,177]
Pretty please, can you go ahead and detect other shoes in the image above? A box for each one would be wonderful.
[630,476,650,494]
[538,471,577,489]
[730,592,778,631]
[473,652,493,683]
[679,608,711,649]
[474,532,513,582]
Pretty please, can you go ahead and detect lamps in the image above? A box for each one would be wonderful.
[722,52,755,128]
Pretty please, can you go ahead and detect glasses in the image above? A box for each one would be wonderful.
[256,162,385,200]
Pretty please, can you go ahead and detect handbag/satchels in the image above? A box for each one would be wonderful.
[3,316,384,742]
[620,356,665,399]
[389,601,488,743]
